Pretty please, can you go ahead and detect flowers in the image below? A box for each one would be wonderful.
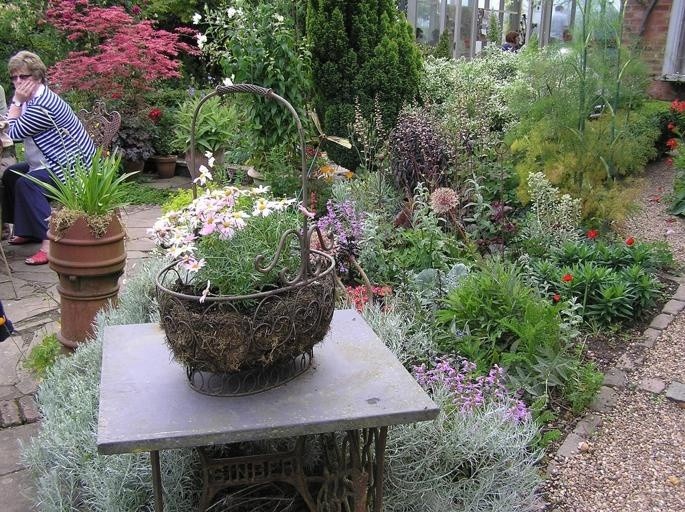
[145,148,318,309]
[138,105,181,157]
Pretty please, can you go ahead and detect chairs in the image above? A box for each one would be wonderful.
[78,102,122,157]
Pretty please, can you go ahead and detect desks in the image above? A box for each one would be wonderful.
[97,308,438,512]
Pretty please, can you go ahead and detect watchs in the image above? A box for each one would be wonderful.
[11,100,23,107]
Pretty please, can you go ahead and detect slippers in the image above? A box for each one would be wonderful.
[8,237,32,244]
[25,251,48,265]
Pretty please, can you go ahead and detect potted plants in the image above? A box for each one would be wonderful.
[7,106,141,349]
[168,92,251,188]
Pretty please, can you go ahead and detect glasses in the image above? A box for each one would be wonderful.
[10,74,32,80]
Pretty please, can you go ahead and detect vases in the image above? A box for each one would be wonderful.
[155,155,177,179]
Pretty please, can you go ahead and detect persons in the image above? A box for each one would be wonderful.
[499,31,521,52]
[0,49,100,266]
[549,5,570,44]
[0,83,20,242]
